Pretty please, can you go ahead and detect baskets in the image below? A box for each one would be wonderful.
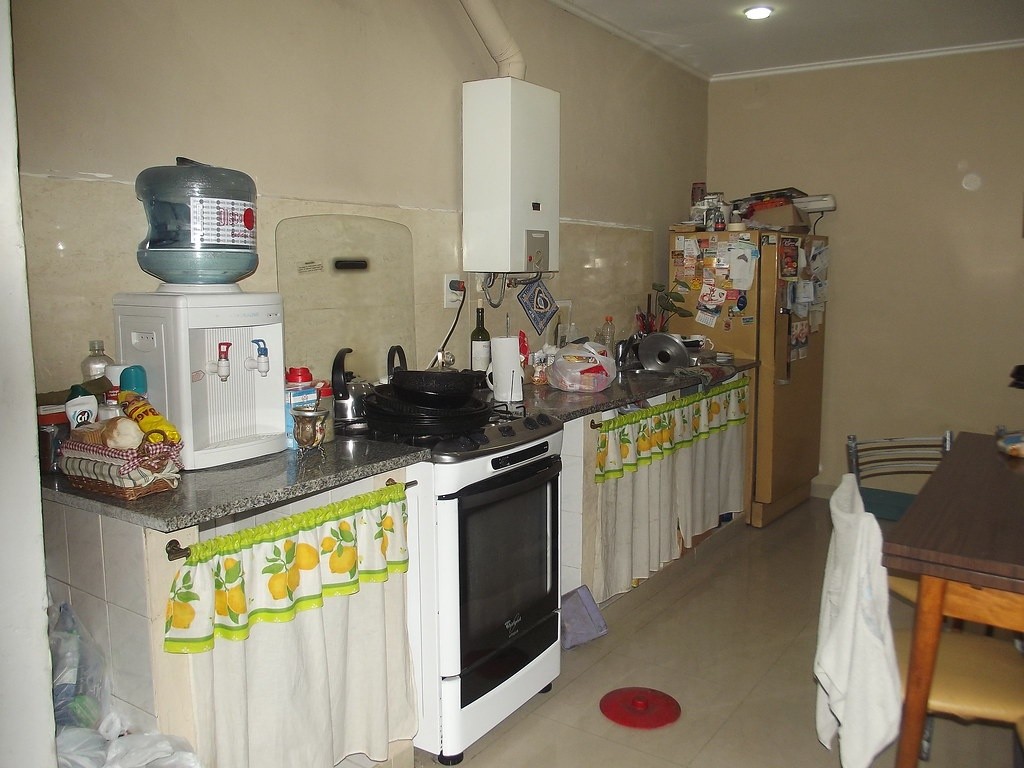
[58,428,183,501]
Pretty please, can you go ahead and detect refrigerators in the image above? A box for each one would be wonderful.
[665,223,830,529]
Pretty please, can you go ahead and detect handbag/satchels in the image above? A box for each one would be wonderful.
[545,341,617,394]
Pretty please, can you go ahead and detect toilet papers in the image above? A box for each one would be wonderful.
[490,335,523,403]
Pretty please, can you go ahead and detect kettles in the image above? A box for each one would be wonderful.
[332,341,375,426]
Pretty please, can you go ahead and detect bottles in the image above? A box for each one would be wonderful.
[287,366,313,387]
[567,321,578,341]
[64,383,99,429]
[133,165,260,284]
[310,378,335,444]
[80,338,114,383]
[99,399,125,422]
[706,205,726,231]
[593,326,605,345]
[602,315,616,359]
[104,362,130,403]
[120,364,148,399]
[730,210,742,223]
[470,307,491,374]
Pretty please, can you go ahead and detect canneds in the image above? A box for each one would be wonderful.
[315,387,335,444]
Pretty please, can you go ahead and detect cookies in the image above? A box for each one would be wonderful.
[70,419,110,446]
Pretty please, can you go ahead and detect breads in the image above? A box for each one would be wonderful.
[101,415,145,450]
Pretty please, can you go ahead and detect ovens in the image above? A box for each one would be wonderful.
[431,411,564,768]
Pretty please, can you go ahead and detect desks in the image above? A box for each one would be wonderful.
[879,431,1024,767]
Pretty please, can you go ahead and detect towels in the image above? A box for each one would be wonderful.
[811,472,908,768]
[671,361,739,397]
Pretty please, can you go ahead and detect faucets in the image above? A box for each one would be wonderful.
[244,339,271,378]
[205,341,233,383]
[615,329,643,366]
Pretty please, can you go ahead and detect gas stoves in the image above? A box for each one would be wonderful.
[366,402,529,449]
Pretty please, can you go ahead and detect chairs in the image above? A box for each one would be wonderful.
[817,471,1024,768]
[845,430,951,498]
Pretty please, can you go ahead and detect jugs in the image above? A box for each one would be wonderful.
[616,339,633,363]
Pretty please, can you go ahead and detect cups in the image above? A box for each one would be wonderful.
[487,354,526,392]
[689,333,714,352]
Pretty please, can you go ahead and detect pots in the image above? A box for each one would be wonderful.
[681,336,704,352]
[361,345,496,437]
